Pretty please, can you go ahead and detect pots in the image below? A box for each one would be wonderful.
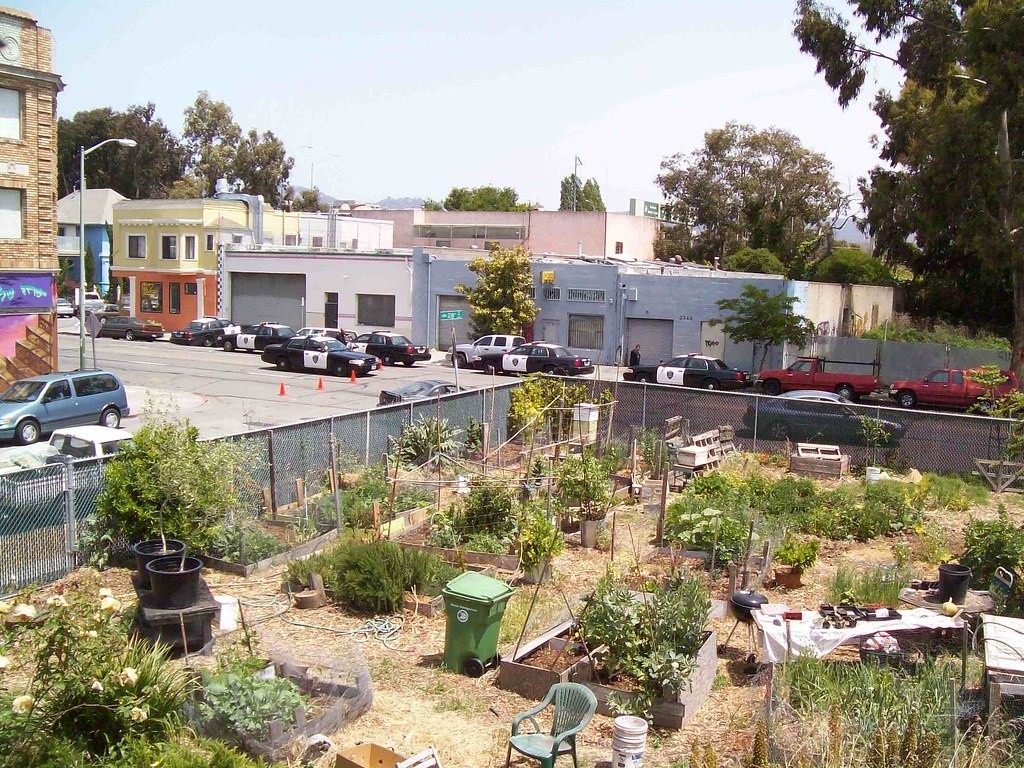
[729,588,770,624]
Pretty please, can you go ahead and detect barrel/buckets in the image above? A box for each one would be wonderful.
[612,716,648,768]
[212,595,238,630]
[865,467,880,485]
[859,649,902,669]
[938,564,973,605]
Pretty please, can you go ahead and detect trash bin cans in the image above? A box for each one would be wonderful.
[631,484,642,503]
[442,570,515,679]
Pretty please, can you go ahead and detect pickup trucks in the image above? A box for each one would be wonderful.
[754,355,882,402]
[889,368,1019,416]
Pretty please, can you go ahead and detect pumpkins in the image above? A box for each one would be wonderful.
[941,596,957,617]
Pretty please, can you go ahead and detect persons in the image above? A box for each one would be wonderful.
[337,329,349,346]
[629,345,641,366]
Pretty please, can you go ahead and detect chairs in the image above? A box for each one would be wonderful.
[506,682,598,766]
[968,567,1013,639]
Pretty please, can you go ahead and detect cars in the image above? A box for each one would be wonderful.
[623,353,754,395]
[0,367,130,446]
[741,389,906,447]
[55,298,74,318]
[260,333,381,377]
[346,329,431,367]
[77,304,130,324]
[97,317,164,342]
[217,322,300,352]
[471,341,596,377]
[446,335,526,368]
[170,316,242,347]
[295,327,358,344]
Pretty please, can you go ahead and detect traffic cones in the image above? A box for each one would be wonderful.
[278,382,288,396]
[316,377,325,390]
[349,370,358,384]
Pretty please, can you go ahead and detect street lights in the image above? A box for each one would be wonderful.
[311,154,340,190]
[280,144,312,209]
[80,138,137,368]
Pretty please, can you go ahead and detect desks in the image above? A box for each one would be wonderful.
[749,607,966,670]
[899,588,995,638]
[978,615,1024,746]
[130,572,222,658]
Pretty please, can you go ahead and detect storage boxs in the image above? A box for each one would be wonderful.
[860,649,917,679]
[677,445,709,467]
[334,744,409,768]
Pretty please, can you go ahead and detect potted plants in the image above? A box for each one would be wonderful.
[556,446,618,548]
[515,509,565,584]
[69,393,270,612]
[772,533,820,589]
